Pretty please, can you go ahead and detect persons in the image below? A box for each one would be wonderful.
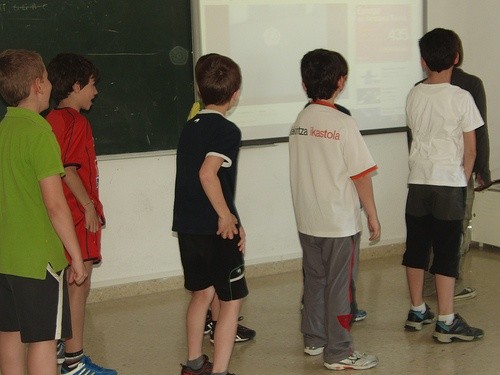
[401,28,492,343]
[289,47,381,371]
[172,53,257,375]
[44,52,118,375]
[0,50,87,375]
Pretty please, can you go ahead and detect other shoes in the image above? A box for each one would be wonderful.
[453,287,478,302]
[353,310,367,321]
[180,354,213,375]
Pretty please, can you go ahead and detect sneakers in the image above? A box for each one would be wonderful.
[208,321,256,343]
[404,304,435,330]
[304,344,326,355]
[324,350,378,370]
[56,341,66,364]
[433,313,484,343]
[61,355,117,375]
[204,318,214,334]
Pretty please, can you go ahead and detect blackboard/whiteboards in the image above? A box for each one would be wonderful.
[0,0,428,161]
